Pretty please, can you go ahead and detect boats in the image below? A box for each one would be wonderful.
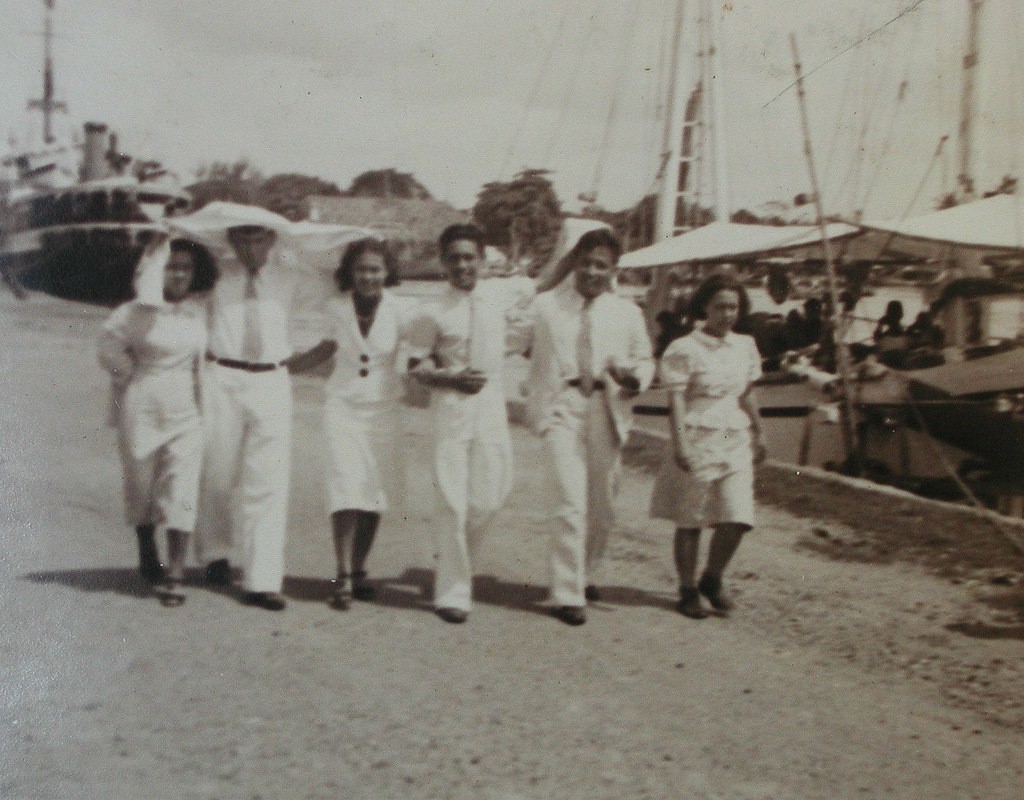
[1,0,225,307]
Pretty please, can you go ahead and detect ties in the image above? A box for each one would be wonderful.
[241,267,263,364]
[576,295,594,397]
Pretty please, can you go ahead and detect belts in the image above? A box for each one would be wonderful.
[205,353,279,372]
[567,377,604,391]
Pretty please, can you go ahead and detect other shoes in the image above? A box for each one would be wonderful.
[140,562,168,596]
[552,604,585,625]
[162,570,185,606]
[438,606,466,623]
[585,585,599,601]
[331,575,352,610]
[351,570,374,600]
[207,558,230,585]
[677,586,706,619]
[242,589,285,610]
[700,576,737,610]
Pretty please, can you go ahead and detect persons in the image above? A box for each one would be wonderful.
[284,234,439,611]
[960,298,989,360]
[93,198,382,613]
[403,221,577,625]
[649,287,837,388]
[658,299,950,373]
[501,226,657,626]
[645,270,769,622]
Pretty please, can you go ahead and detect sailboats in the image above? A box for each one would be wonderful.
[604,0,1022,517]
